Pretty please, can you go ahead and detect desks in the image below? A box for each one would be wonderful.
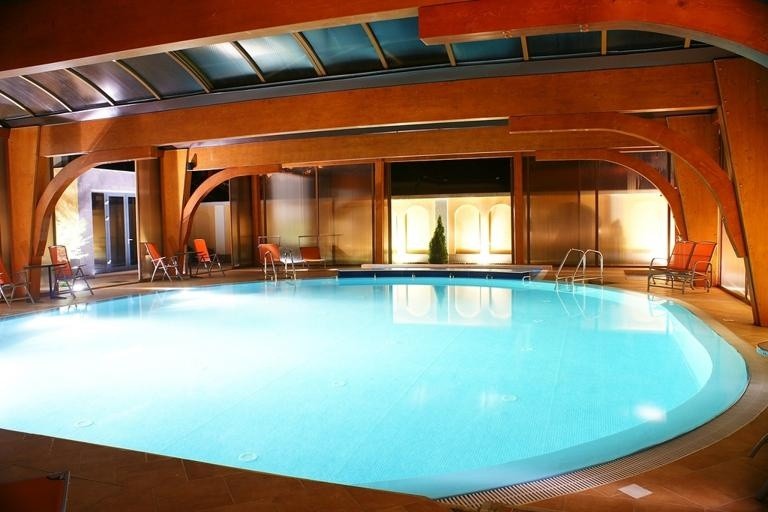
[175,250,204,281]
[21,261,66,299]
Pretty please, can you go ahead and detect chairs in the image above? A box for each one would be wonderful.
[0,257,37,307]
[298,247,327,269]
[668,241,718,293]
[145,243,184,282]
[649,242,695,294]
[191,238,225,278]
[255,243,283,273]
[48,246,94,298]
